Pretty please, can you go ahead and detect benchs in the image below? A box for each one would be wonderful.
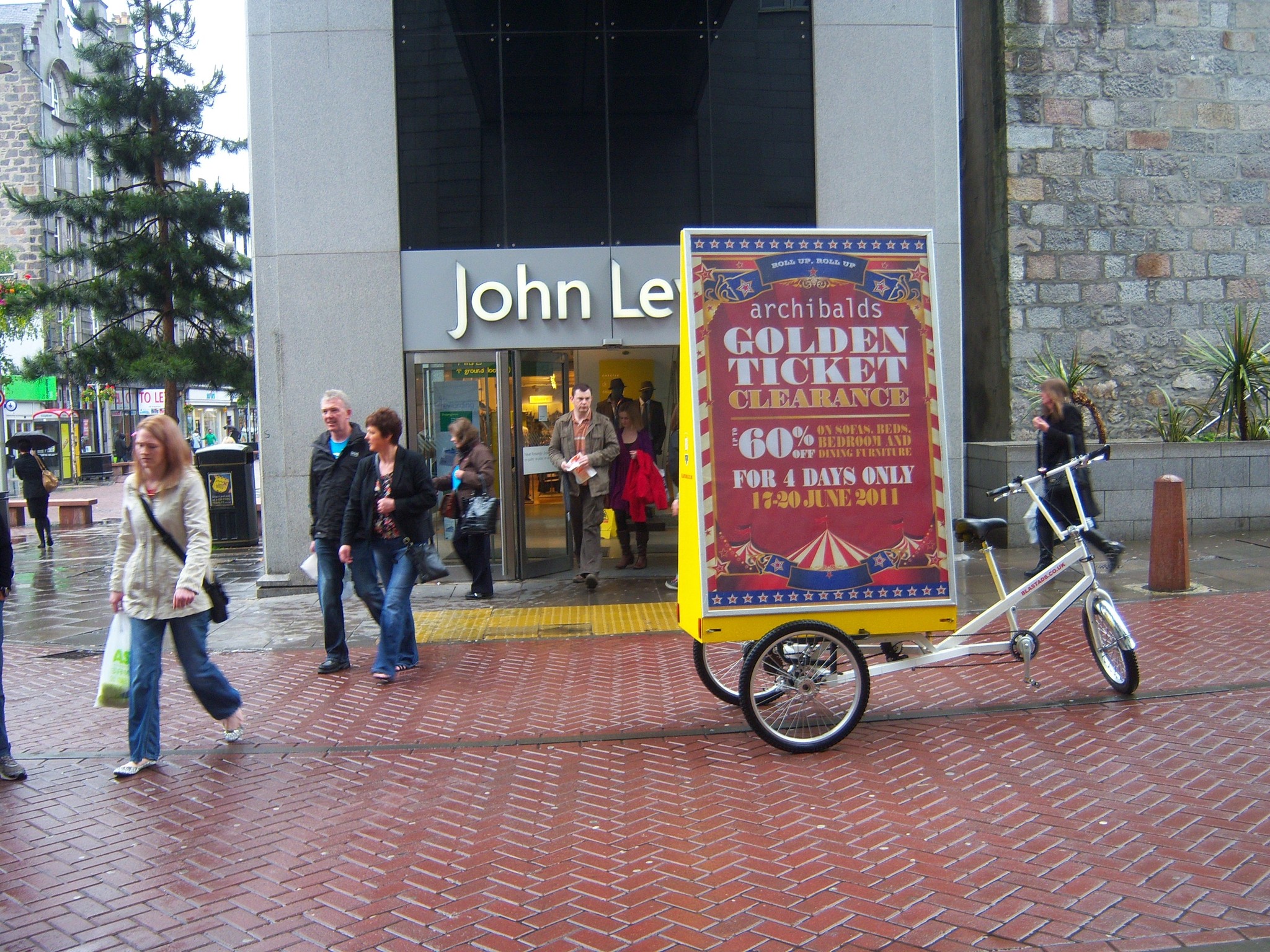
[8,498,98,527]
[112,461,135,475]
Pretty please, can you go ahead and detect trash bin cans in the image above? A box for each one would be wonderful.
[194,444,259,549]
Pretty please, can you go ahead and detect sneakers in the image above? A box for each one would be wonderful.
[665,574,678,589]
[0,755,26,781]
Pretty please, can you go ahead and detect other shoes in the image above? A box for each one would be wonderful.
[38,543,45,548]
[113,760,156,775]
[585,574,598,589]
[525,496,533,501]
[465,593,493,599]
[224,723,244,742]
[47,538,53,546]
[573,573,585,582]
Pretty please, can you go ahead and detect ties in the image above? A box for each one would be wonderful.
[642,403,648,428]
[613,404,619,427]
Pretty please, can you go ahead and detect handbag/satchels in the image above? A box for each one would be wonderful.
[440,492,458,519]
[1048,467,1098,519]
[456,474,499,534]
[34,455,59,493]
[406,541,450,583]
[93,607,132,708]
[205,580,229,623]
[300,552,355,601]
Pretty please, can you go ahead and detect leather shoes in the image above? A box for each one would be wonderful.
[318,661,350,673]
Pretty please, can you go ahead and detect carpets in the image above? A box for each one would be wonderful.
[630,543,678,554]
[555,555,678,580]
[443,546,610,559]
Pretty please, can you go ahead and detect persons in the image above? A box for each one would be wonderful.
[511,420,534,503]
[431,417,494,599]
[664,493,679,589]
[668,404,679,487]
[192,429,200,454]
[602,400,652,569]
[115,433,129,463]
[14,442,53,548]
[1024,378,1126,578]
[309,389,386,674]
[201,429,218,446]
[110,416,242,775]
[547,383,620,588]
[230,427,241,443]
[597,378,666,465]
[338,409,439,680]
[0,518,27,780]
[128,435,136,451]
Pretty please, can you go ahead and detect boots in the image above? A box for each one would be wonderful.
[1082,527,1126,573]
[1024,523,1054,577]
[634,531,649,569]
[614,529,634,568]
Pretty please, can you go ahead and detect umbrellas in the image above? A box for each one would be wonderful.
[223,425,237,430]
[5,432,58,451]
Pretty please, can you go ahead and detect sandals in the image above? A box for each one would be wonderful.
[394,664,409,671]
[373,671,393,681]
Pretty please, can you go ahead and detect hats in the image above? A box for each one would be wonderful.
[638,381,656,392]
[607,378,627,390]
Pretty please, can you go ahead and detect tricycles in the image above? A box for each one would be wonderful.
[692,444,1142,753]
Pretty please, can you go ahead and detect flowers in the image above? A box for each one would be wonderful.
[81,384,116,405]
[0,274,36,333]
